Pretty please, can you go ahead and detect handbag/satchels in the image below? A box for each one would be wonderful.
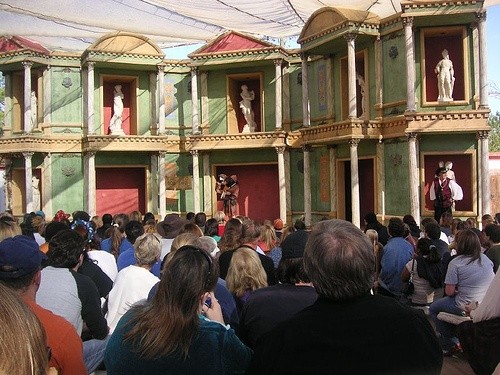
[400,281,415,293]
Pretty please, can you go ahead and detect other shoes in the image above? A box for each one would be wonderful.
[443,342,462,357]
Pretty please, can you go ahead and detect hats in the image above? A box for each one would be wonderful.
[273,218,283,229]
[364,212,376,224]
[52,210,70,224]
[195,213,206,227]
[282,231,309,257]
[157,214,187,239]
[0,234,49,279]
[35,211,45,218]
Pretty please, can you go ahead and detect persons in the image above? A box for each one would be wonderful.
[215,174,228,201]
[356,74,365,114]
[0,211,500,375]
[430,166,463,224]
[238,85,258,133]
[31,90,38,128]
[434,49,455,101]
[32,175,40,213]
[444,161,456,211]
[109,85,126,135]
[221,174,240,226]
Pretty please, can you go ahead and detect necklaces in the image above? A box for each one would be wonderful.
[228,183,236,189]
[438,178,447,191]
